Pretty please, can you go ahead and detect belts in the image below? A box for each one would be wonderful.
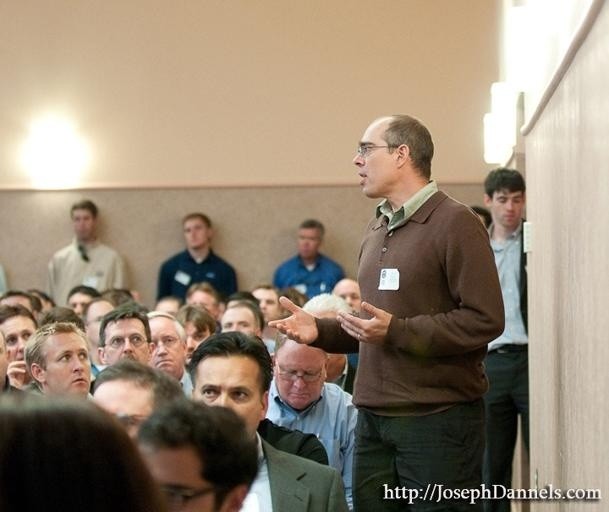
[489,340,529,355]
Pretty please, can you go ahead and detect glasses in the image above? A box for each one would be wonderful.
[273,355,326,384]
[357,144,399,157]
[155,483,236,509]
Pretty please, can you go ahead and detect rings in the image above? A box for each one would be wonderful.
[358,334,361,343]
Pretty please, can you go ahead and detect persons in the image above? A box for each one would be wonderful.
[467,204,491,230]
[46,199,131,310]
[272,217,342,300]
[473,167,528,510]
[154,211,239,303]
[265,112,507,511]
